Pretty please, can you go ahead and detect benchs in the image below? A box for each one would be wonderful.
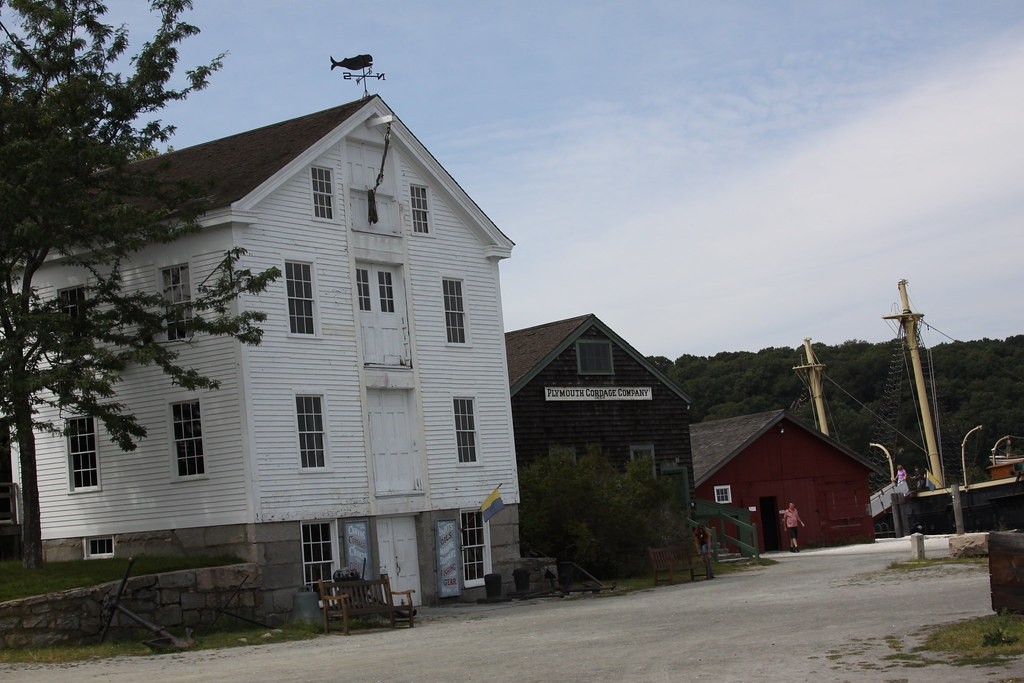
[317,573,416,637]
[647,542,709,585]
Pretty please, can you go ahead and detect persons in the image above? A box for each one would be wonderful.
[910,466,924,491]
[784,502,805,552]
[695,524,716,580]
[893,465,909,496]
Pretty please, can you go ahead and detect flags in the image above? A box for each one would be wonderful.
[926,470,937,491]
[480,488,505,522]
